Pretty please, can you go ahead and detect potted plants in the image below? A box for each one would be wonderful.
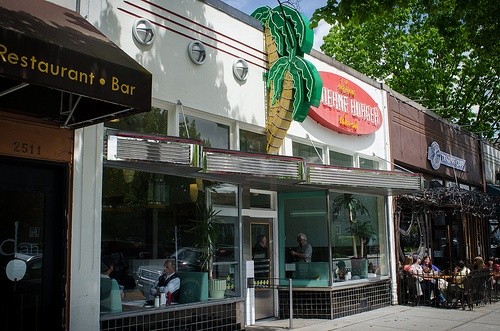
[185,203,226,299]
[331,193,377,279]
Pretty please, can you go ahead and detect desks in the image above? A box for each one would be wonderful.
[424,276,452,290]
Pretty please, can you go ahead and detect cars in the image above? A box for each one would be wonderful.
[15,253,65,298]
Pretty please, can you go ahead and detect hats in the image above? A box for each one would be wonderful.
[336,261,346,267]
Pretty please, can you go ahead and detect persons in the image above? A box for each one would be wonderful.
[253,234,269,282]
[290,232,312,263]
[100,254,125,313]
[403,254,500,306]
[150,258,181,302]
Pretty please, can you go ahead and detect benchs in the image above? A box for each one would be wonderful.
[293,262,329,279]
[100,278,121,315]
[171,272,209,304]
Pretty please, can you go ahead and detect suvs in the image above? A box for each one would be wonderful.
[134,244,238,305]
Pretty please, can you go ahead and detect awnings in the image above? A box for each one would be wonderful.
[0,0,153,131]
